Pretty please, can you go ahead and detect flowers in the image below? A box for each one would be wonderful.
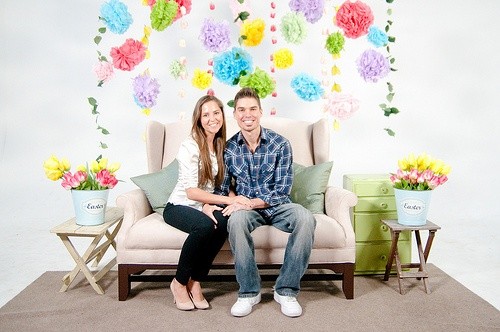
[43,154,120,190]
[390,153,451,190]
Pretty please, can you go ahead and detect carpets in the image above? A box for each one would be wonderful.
[0,261,500,332]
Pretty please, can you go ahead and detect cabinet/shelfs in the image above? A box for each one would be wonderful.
[343,172,412,275]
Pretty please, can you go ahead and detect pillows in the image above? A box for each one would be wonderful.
[130,158,179,216]
[289,161,333,214]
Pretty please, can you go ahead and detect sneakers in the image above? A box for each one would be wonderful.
[272,290,302,317]
[230,291,262,317]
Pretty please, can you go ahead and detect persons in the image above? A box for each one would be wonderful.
[212,87,316,319]
[163,95,254,311]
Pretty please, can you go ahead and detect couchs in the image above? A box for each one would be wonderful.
[113,119,356,300]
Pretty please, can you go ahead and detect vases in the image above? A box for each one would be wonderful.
[71,188,108,225]
[394,188,431,226]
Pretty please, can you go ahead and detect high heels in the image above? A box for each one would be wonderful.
[188,290,210,310]
[170,279,195,311]
